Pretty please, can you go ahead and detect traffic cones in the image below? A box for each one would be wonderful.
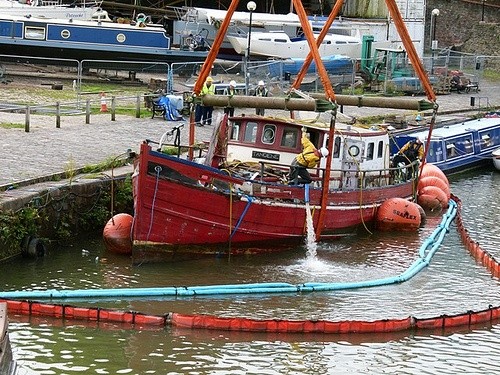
[99,92,108,112]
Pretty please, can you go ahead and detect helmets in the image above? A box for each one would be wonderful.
[206,76,214,82]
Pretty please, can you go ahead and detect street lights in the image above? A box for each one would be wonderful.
[431,8,440,74]
[245,2,256,95]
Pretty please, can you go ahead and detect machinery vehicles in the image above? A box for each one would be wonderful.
[354,34,414,83]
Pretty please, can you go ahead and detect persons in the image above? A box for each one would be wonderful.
[452,73,463,94]
[223,81,239,118]
[252,81,270,116]
[392,137,425,181]
[194,76,217,126]
[290,128,328,186]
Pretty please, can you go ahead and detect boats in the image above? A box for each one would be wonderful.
[226,32,361,59]
[389,117,500,176]
[129,107,422,267]
[0,0,210,71]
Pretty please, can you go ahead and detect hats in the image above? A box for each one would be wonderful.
[229,80,237,88]
[418,135,426,143]
[320,146,329,158]
[258,80,265,86]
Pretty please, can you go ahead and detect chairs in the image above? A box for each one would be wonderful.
[181,91,194,117]
[151,96,168,120]
